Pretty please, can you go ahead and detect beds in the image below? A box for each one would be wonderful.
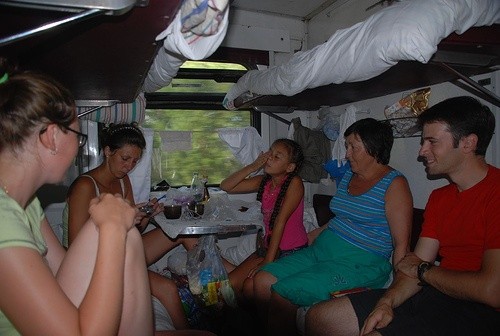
[239,194,443,336]
[0,0,232,126]
[221,0,500,133]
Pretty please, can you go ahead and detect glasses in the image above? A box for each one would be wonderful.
[38,125,89,149]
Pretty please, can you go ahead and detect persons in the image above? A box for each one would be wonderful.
[305,96,500,336]
[219,138,310,307]
[256,118,413,336]
[62,122,199,330]
[0,56,216,336]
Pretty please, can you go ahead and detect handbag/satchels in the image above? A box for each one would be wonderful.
[187,234,236,304]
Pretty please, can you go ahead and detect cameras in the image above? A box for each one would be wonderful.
[138,205,154,215]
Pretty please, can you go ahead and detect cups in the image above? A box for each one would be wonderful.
[164,205,182,219]
[188,204,205,217]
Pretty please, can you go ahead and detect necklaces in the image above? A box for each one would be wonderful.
[0,182,9,193]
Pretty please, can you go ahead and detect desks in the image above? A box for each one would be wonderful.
[151,209,266,239]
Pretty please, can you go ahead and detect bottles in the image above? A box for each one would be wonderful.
[219,274,237,306]
[199,267,218,308]
[190,173,202,201]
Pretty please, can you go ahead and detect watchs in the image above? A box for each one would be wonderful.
[417,261,432,286]
